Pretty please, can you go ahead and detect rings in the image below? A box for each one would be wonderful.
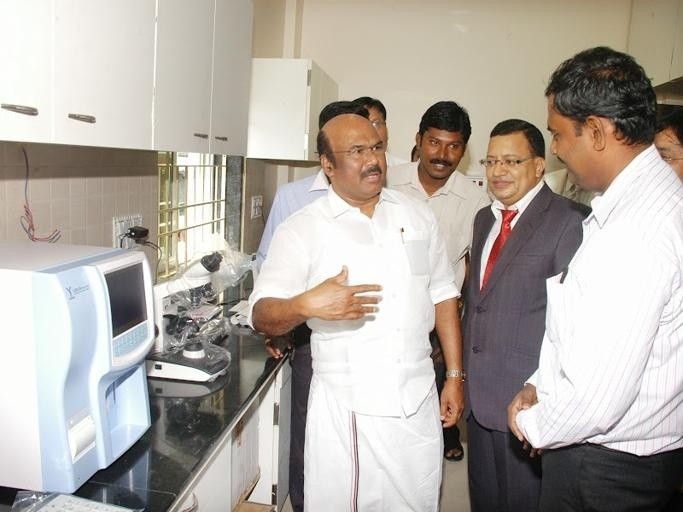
[264,338,271,344]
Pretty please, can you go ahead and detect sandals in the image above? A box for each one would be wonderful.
[441,422,464,462]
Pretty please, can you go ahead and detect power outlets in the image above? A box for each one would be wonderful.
[250,194,262,221]
[112,215,145,251]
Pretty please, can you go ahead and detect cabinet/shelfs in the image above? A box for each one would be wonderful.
[247,55,341,164]
[620,0,682,105]
[1,0,154,158]
[152,1,254,158]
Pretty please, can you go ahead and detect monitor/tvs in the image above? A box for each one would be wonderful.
[85,251,155,371]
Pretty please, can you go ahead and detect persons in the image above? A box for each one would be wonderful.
[411,145,421,162]
[462,119,590,512]
[254,102,369,512]
[388,100,490,459]
[506,46,683,512]
[248,115,467,512]
[545,167,603,211]
[656,105,683,187]
[355,97,406,166]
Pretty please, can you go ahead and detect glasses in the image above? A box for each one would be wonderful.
[368,120,386,128]
[312,141,388,159]
[658,153,683,165]
[477,154,540,171]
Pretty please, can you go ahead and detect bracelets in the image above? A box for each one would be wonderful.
[444,369,464,378]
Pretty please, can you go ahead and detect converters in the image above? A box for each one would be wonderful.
[128,226,148,237]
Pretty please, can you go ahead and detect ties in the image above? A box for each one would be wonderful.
[477,207,521,294]
[569,183,582,206]
